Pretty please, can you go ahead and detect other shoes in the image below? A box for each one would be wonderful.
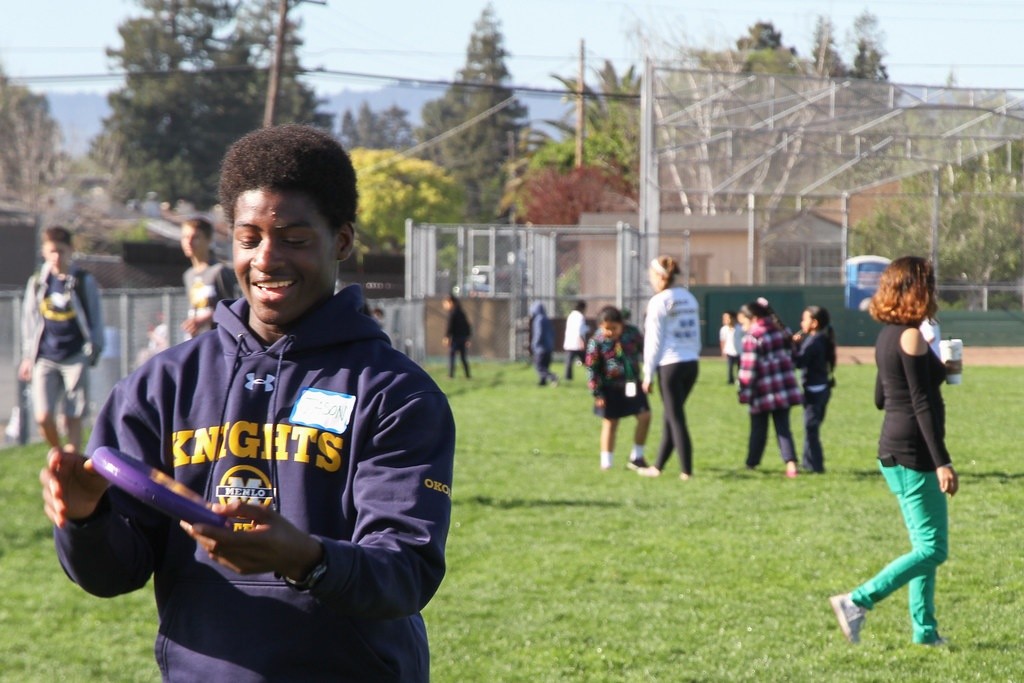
[788,470,798,478]
[627,458,646,470]
[680,473,689,480]
[637,466,660,477]
[829,593,866,646]
[925,637,948,647]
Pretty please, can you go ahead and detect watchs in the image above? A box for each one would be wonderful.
[283,546,328,593]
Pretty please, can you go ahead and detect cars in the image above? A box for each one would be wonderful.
[451,264,508,296]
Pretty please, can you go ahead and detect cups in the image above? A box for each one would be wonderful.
[940,339,963,384]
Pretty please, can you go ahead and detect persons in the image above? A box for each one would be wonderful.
[181,217,236,337]
[792,305,836,473]
[20,227,105,454]
[532,304,560,386]
[563,301,652,469]
[640,257,700,479]
[39,124,456,683]
[830,256,959,648]
[920,315,942,360]
[443,295,471,378]
[737,298,804,478]
[720,312,744,384]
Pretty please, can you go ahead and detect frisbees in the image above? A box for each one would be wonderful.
[91,446,227,528]
